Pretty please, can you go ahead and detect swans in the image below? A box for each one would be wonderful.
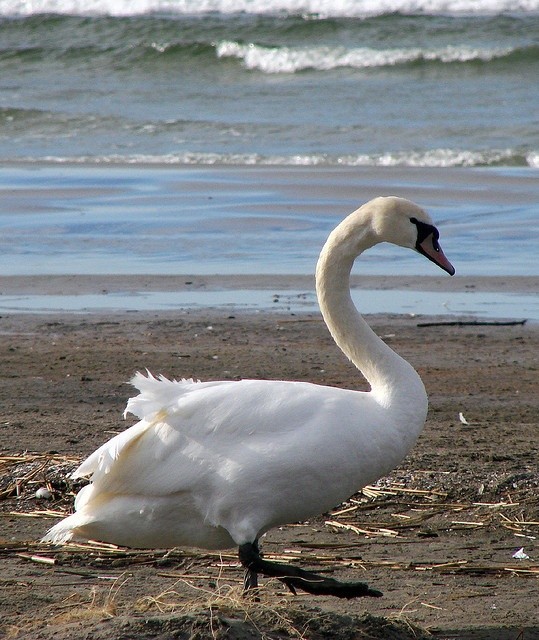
[38,195,456,603]
